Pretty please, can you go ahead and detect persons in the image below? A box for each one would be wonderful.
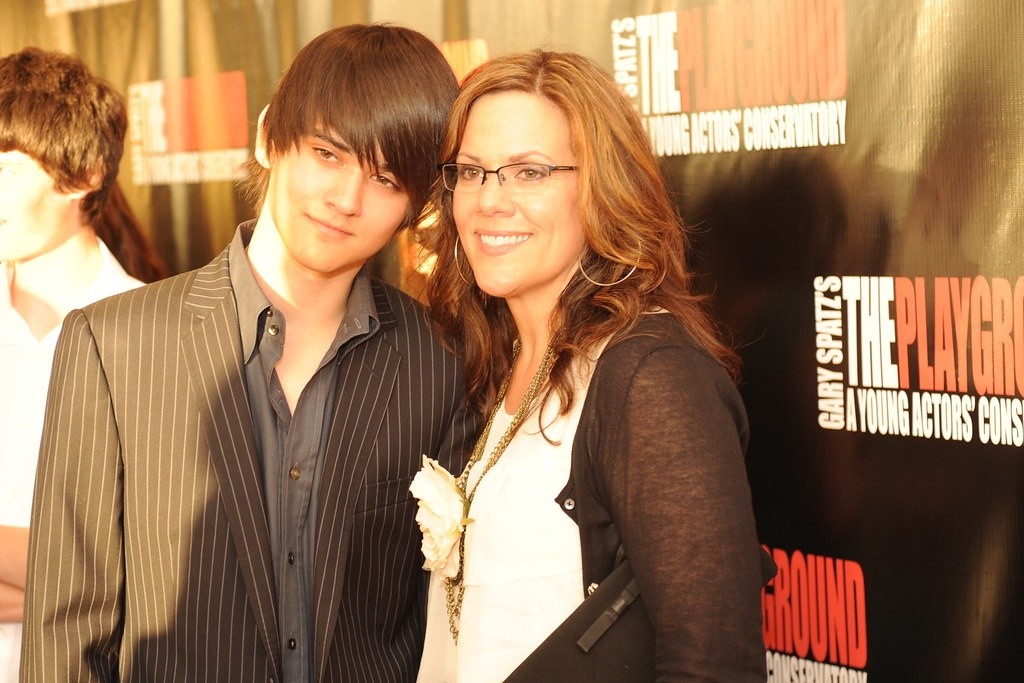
[15,24,489,682]
[1,45,150,683]
[411,46,769,682]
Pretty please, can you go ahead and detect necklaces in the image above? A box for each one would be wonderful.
[408,325,562,646]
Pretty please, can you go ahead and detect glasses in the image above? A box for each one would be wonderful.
[440,161,581,194]
